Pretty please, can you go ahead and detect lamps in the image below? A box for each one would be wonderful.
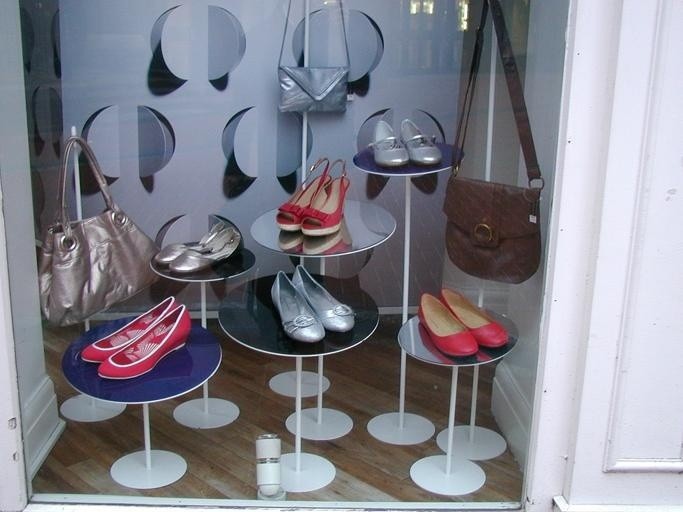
[254,433,286,501]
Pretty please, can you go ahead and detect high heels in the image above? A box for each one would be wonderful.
[301,159,349,236]
[274,159,332,233]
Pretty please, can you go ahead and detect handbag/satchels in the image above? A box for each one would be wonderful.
[277,66,348,113]
[37,208,161,328]
[442,176,541,285]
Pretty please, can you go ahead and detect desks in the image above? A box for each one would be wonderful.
[61,318,223,492]
[250,199,397,441]
[151,246,254,430]
[352,141,466,448]
[402,301,519,496]
[218,273,381,492]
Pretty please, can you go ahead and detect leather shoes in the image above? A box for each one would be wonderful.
[97,304,190,380]
[154,222,226,266]
[81,296,177,362]
[270,270,324,342]
[169,227,241,272]
[417,292,478,357]
[368,121,408,167]
[399,119,442,166]
[292,264,354,333]
[439,288,508,347]
